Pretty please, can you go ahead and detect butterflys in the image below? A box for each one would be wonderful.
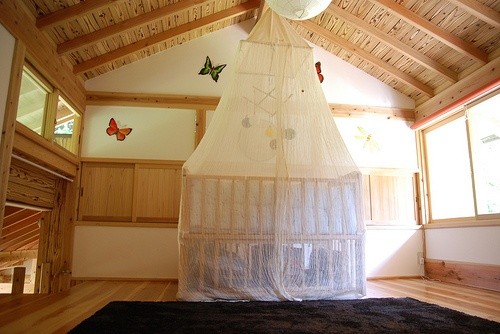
[315,61,324,83]
[198,56,227,83]
[106,118,132,141]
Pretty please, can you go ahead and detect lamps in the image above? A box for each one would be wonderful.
[266,0,332,21]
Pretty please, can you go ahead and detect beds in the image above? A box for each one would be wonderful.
[179,165,366,299]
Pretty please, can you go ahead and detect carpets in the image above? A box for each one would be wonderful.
[63,296,500,334]
